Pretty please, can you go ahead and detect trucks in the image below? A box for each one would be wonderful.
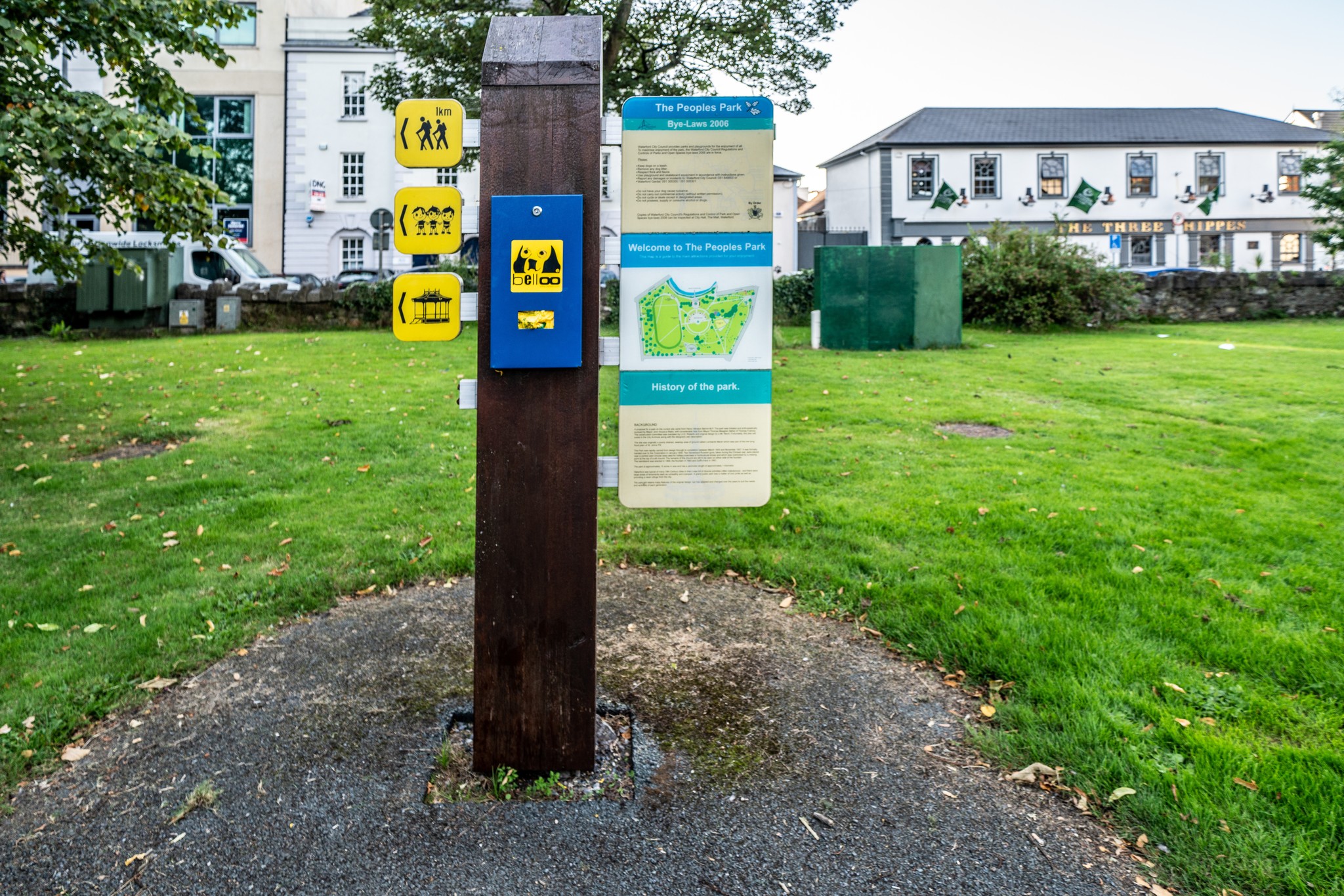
[26,228,305,294]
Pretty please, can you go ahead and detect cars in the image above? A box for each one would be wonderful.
[295,272,322,291]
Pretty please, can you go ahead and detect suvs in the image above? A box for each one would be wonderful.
[335,269,395,290]
